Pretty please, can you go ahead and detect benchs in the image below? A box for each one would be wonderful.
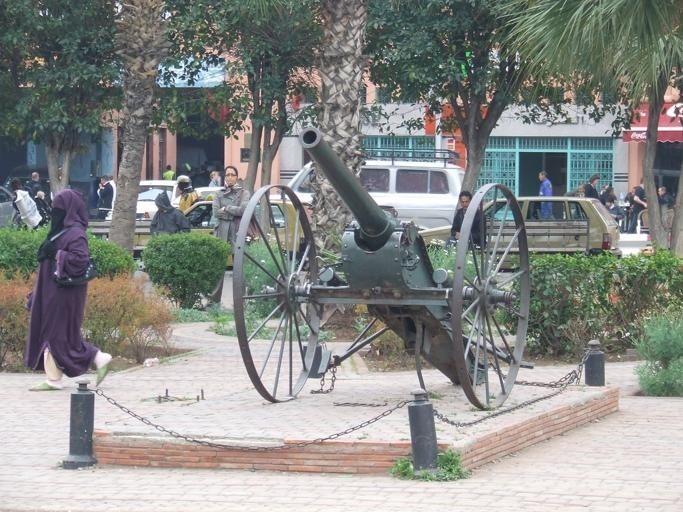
[475,219,592,260]
[85,221,157,258]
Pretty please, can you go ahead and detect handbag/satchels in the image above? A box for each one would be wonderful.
[235,214,260,246]
[49,249,99,287]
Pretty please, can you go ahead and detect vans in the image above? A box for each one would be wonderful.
[269,148,466,225]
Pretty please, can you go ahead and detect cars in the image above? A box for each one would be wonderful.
[417,196,621,258]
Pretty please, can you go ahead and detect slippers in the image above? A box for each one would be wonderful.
[29,382,63,391]
[95,359,113,387]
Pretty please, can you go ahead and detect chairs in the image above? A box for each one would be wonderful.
[636,209,649,237]
[662,209,675,231]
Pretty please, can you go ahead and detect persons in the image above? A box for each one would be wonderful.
[534,166,552,220]
[8,161,250,257]
[24,188,113,391]
[448,192,484,247]
[560,171,673,235]
